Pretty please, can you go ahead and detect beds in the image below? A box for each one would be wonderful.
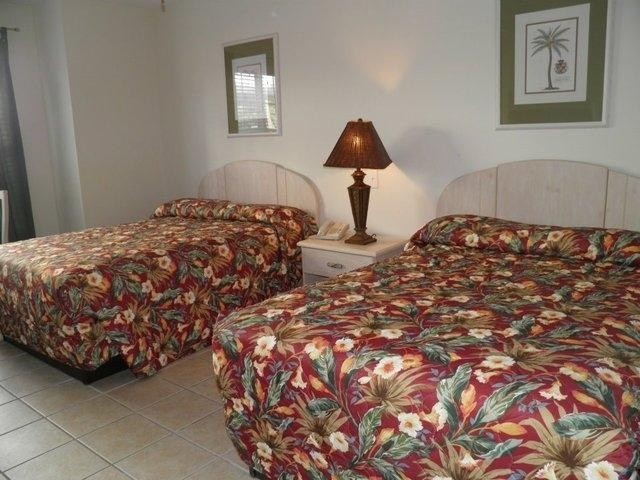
[0,161,319,386]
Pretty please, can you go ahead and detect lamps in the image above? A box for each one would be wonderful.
[322,117,394,244]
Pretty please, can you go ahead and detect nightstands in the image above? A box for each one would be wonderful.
[295,233,407,286]
[210,157,640,478]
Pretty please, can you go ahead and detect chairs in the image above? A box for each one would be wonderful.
[0,188,10,244]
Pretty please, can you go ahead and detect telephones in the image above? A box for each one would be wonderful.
[316,216,350,241]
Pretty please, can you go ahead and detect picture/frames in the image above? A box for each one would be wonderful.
[495,0,618,130]
[222,32,282,140]
